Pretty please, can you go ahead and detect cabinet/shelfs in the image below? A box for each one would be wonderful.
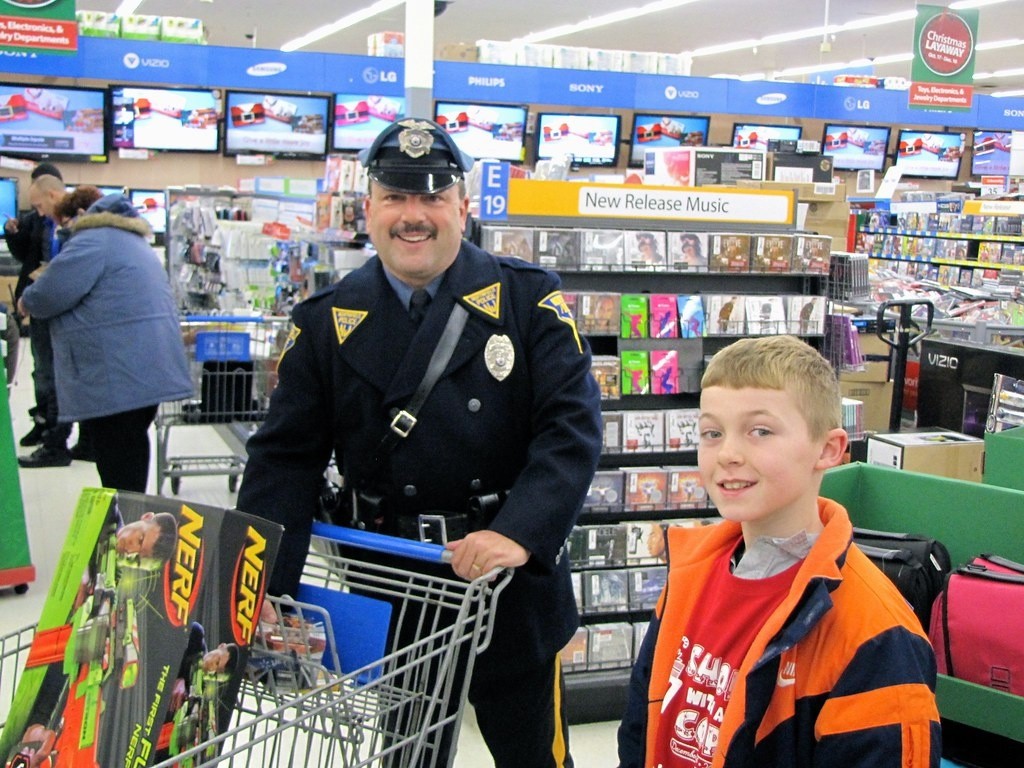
[300,193,1024,728]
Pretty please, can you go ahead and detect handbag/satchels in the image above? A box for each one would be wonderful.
[927,553,1023,698]
[851,525,951,642]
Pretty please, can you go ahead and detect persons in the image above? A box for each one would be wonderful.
[4,165,65,445]
[18,173,100,468]
[73,493,238,728]
[16,186,190,493]
[234,117,604,768]
[618,334,942,768]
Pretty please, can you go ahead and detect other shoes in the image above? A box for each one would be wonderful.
[16,446,73,468]
[19,417,59,446]
[69,442,97,463]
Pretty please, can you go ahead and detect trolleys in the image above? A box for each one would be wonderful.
[153,304,288,494]
[1,517,517,768]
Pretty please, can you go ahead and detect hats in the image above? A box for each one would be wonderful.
[356,118,476,196]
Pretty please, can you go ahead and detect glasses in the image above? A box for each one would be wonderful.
[61,216,71,229]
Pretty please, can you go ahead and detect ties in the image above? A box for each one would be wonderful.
[409,290,429,325]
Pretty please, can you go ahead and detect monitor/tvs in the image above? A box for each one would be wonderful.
[65,184,166,234]
[0,83,405,163]
[435,100,1012,182]
[0,176,20,238]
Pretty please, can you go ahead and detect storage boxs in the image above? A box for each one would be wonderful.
[0,484,288,768]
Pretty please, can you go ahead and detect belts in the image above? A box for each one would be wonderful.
[337,485,511,547]
